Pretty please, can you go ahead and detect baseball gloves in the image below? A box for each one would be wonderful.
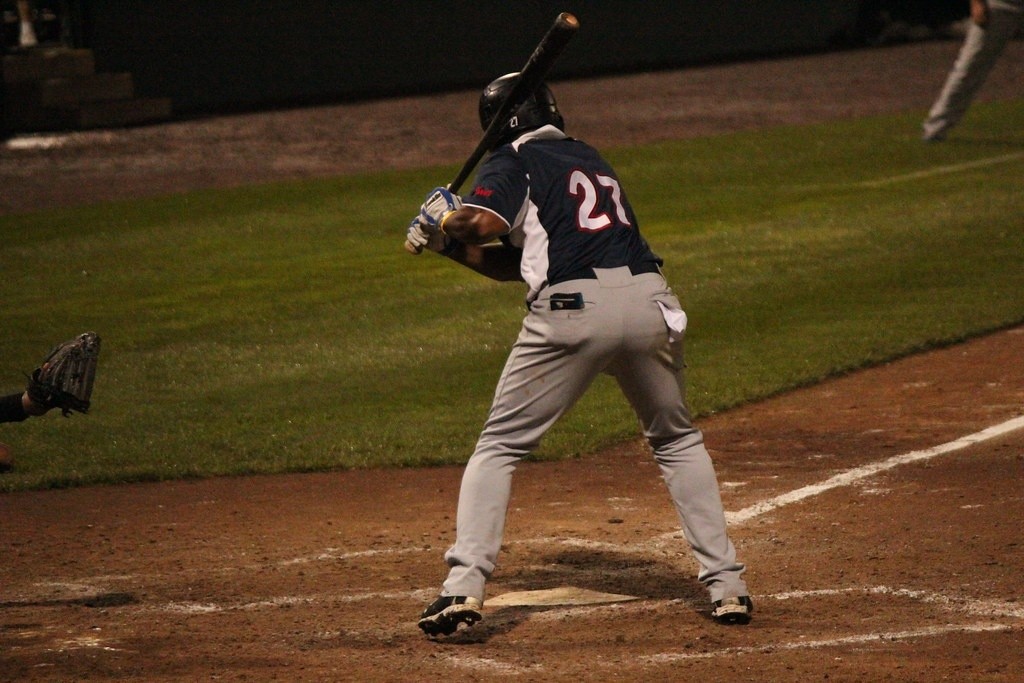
[28,329,100,413]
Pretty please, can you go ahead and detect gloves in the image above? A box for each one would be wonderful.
[404,214,459,258]
[420,183,463,237]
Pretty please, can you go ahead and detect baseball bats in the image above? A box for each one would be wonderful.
[421,11,579,226]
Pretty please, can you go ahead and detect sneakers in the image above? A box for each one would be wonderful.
[711,594,754,624]
[418,594,483,640]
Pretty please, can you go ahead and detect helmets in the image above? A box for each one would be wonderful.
[478,72,566,152]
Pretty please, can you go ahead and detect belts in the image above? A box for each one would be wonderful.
[548,262,664,288]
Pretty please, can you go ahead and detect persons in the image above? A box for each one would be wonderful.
[922,0,1024,142]
[406,73,752,641]
